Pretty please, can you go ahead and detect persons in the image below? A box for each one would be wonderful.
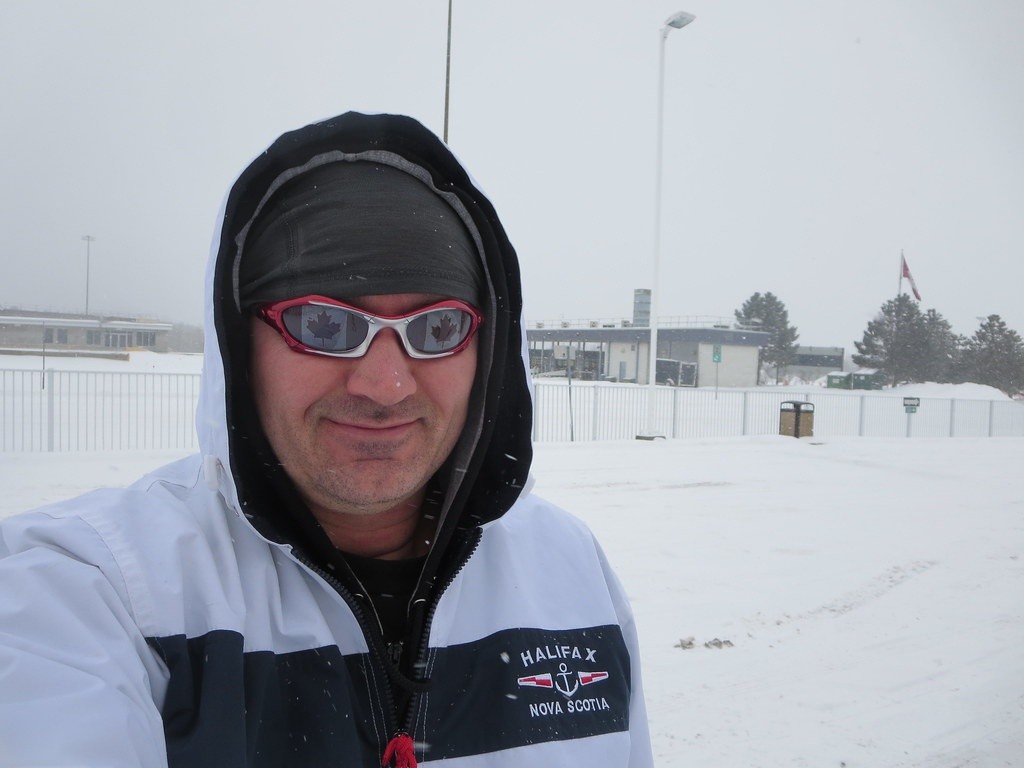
[0,112,654,768]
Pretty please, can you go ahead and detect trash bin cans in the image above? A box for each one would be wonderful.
[779,400,814,438]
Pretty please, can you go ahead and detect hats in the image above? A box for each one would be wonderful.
[238,160,491,310]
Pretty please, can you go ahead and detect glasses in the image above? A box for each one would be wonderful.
[254,294,485,360]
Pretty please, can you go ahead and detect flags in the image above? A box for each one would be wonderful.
[903,257,921,301]
